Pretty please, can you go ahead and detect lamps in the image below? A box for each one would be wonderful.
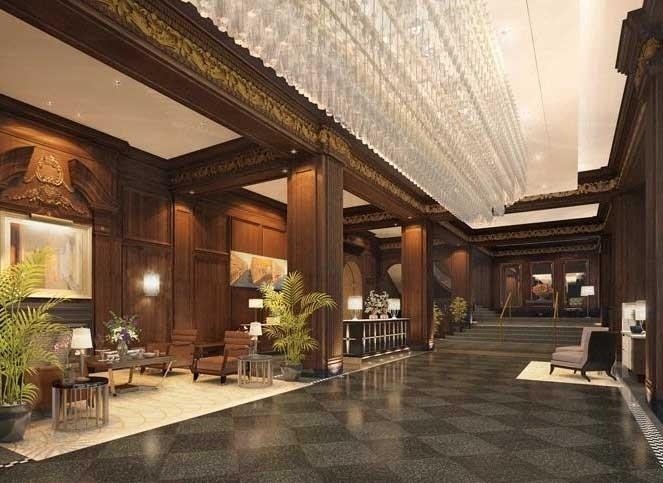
[388,299,402,321]
[71,327,93,382]
[248,298,264,321]
[634,300,645,331]
[249,322,263,356]
[143,272,161,297]
[348,296,363,321]
[30,214,74,232]
[580,286,595,319]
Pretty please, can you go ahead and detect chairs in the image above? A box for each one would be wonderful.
[556,326,610,375]
[549,331,618,382]
[141,329,198,378]
[193,331,253,383]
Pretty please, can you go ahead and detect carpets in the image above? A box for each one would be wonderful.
[0,368,312,461]
[516,360,625,387]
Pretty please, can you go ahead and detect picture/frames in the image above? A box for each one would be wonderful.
[380,314,389,319]
[0,210,92,300]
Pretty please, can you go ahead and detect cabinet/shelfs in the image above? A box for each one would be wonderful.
[621,331,645,382]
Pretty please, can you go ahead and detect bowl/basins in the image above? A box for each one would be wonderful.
[142,353,155,358]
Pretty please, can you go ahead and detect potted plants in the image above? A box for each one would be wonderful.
[0,245,72,443]
[257,270,338,381]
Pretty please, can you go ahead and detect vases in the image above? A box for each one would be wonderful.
[369,315,378,320]
[117,341,129,360]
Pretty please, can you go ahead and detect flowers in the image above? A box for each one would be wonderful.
[103,310,142,344]
[364,289,383,314]
[379,291,391,314]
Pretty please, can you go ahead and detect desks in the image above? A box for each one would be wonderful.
[344,318,410,360]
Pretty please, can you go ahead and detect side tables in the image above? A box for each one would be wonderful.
[52,377,108,433]
[237,354,273,387]
